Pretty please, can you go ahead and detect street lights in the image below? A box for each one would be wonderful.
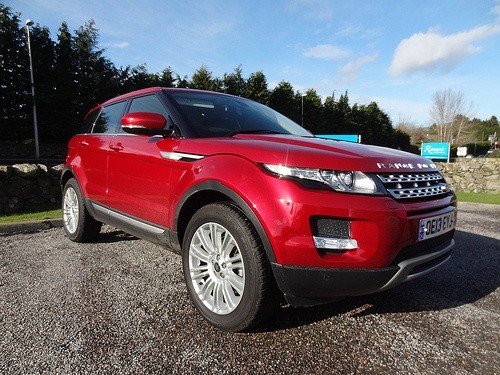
[25,18,41,159]
[301,94,308,128]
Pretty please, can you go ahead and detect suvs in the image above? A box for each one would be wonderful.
[60,85,459,334]
